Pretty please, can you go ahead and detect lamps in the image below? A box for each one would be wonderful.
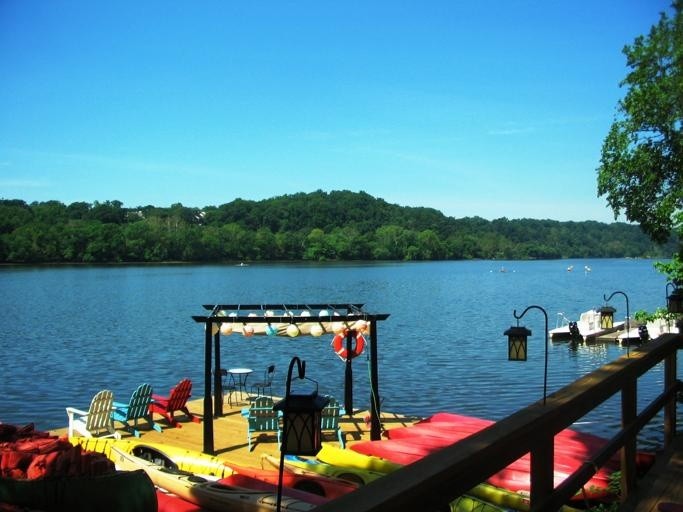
[274,356,332,511]
[596,291,629,359]
[503,305,549,409]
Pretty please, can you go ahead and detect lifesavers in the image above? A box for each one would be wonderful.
[333,329,364,359]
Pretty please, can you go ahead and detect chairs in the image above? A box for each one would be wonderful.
[65,377,200,440]
[212,364,275,409]
[242,395,346,454]
